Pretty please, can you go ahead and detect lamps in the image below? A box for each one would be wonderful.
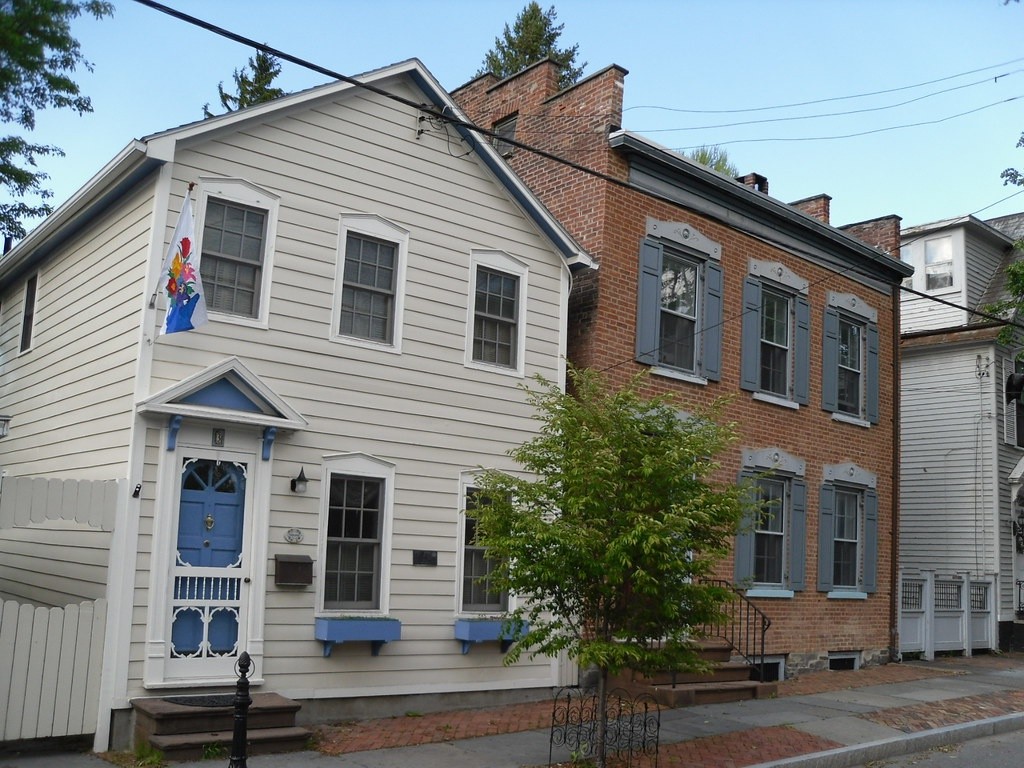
[291,465,310,494]
[1013,509,1024,536]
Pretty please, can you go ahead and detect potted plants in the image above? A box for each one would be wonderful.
[455,617,530,641]
[314,616,402,642]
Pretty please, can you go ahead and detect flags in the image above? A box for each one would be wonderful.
[158,194,207,336]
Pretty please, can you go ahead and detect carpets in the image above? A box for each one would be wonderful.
[163,694,253,708]
